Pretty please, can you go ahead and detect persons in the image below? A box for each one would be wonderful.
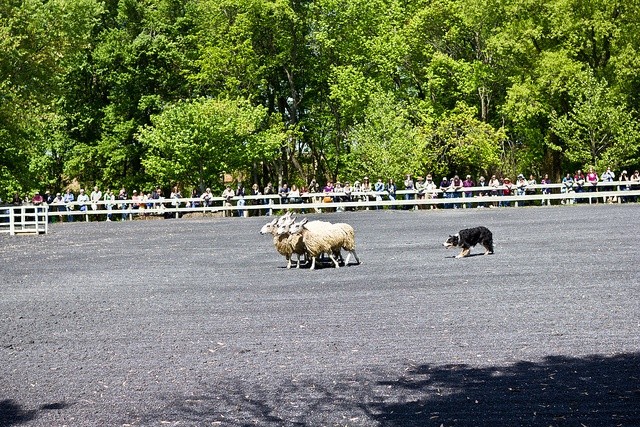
[222,186,235,217]
[263,181,275,216]
[498,177,504,207]
[600,167,615,204]
[403,174,413,210]
[310,179,320,212]
[586,167,599,204]
[527,174,537,206]
[386,178,397,210]
[236,183,245,216]
[191,188,200,211]
[344,181,355,211]
[374,179,385,209]
[489,175,499,207]
[619,170,630,202]
[119,188,128,220]
[169,187,182,218]
[300,185,309,214]
[560,172,576,204]
[44,191,52,222]
[362,176,371,210]
[11,194,21,220]
[131,188,139,216]
[477,176,486,208]
[22,196,33,220]
[334,181,346,212]
[516,173,529,207]
[353,181,361,211]
[322,181,334,213]
[287,184,302,214]
[199,188,213,212]
[53,192,66,221]
[278,183,290,213]
[439,177,450,208]
[76,189,90,221]
[412,175,424,210]
[449,178,454,208]
[89,185,103,221]
[138,188,167,217]
[463,175,475,208]
[540,174,552,206]
[572,169,585,204]
[32,192,42,221]
[502,177,513,207]
[63,188,74,221]
[250,184,263,217]
[424,174,438,209]
[630,169,640,203]
[103,187,112,222]
[110,192,117,207]
[451,174,463,209]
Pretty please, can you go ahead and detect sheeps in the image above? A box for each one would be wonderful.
[260,210,362,270]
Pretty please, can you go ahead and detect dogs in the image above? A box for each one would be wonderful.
[442,225,494,258]
